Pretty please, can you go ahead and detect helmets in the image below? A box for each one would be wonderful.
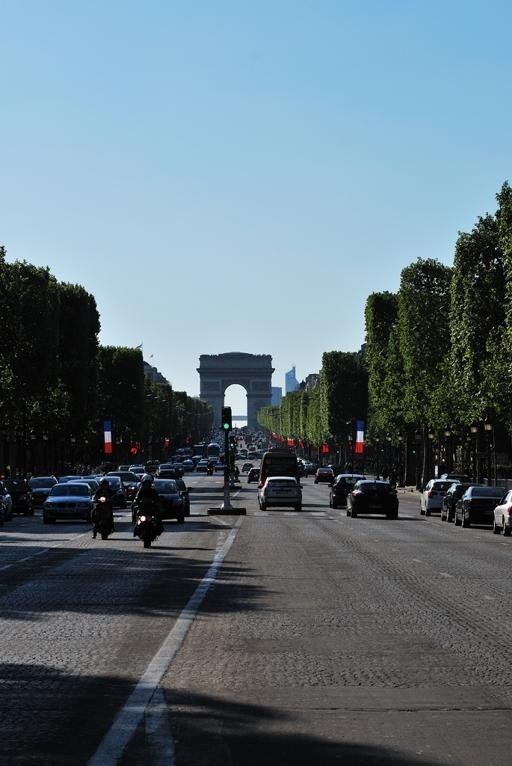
[140,473,154,483]
[98,477,109,490]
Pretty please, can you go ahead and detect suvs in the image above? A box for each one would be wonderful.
[419,478,461,516]
[345,479,399,520]
[327,473,366,509]
[258,476,304,512]
[440,482,484,522]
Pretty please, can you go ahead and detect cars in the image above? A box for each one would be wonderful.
[0,427,226,523]
[441,473,472,483]
[229,425,277,483]
[493,489,512,536]
[298,459,345,484]
[454,486,507,528]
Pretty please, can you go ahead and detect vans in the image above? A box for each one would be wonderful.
[256,448,303,489]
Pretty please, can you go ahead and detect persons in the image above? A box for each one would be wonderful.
[131,473,166,537]
[90,478,115,539]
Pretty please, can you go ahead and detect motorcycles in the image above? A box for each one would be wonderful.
[87,493,124,541]
[127,494,166,549]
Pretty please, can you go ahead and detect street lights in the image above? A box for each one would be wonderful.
[470,420,480,481]
[397,428,405,487]
[426,425,436,477]
[375,432,381,480]
[483,414,495,486]
[414,426,424,489]
[444,423,453,471]
[385,429,394,472]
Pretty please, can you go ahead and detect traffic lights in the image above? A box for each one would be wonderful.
[221,407,232,432]
